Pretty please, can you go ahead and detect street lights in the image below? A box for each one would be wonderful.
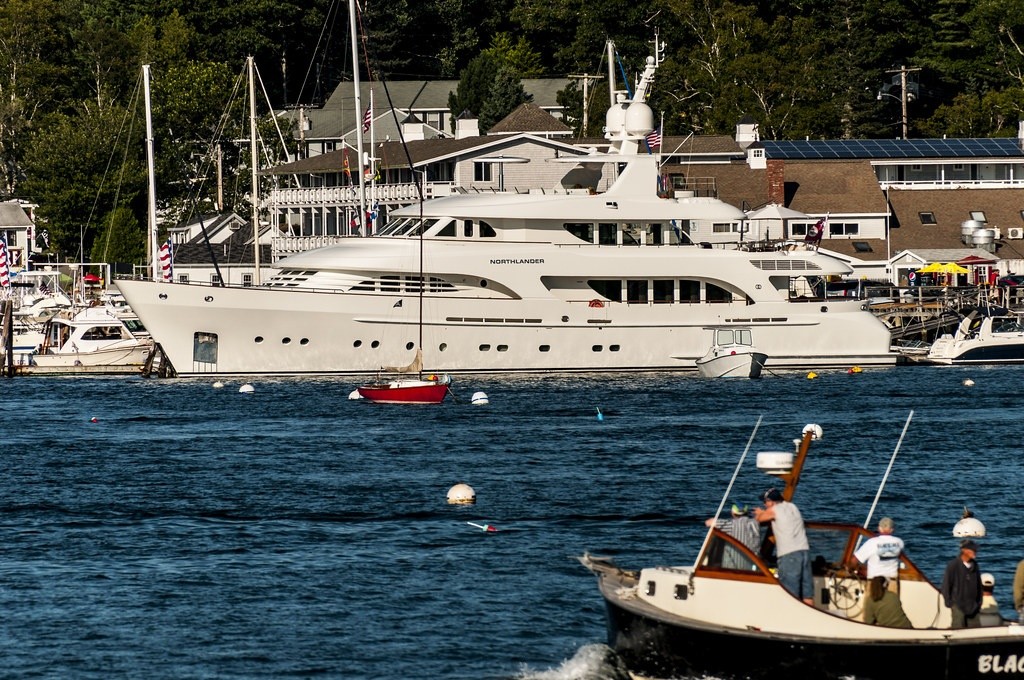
[876,90,917,140]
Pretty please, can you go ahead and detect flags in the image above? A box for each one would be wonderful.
[361,104,371,135]
[0,233,10,289]
[35,230,51,251]
[38,281,52,295]
[342,144,378,227]
[804,219,822,242]
[159,237,173,283]
[646,128,660,148]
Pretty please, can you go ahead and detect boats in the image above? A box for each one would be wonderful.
[579,409,1024,679]
[2,1,1021,380]
[695,327,769,379]
[348,349,454,404]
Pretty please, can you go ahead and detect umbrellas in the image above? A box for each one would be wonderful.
[83,273,103,280]
[59,274,72,282]
[915,263,972,274]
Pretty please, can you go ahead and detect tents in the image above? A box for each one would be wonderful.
[956,255,997,265]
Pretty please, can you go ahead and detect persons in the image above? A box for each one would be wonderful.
[862,575,912,630]
[704,504,760,573]
[990,269,1001,305]
[754,487,815,606]
[1014,559,1024,625]
[850,517,904,600]
[942,541,983,629]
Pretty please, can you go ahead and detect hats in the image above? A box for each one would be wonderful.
[980,572,996,586]
[960,539,977,550]
[732,503,748,518]
